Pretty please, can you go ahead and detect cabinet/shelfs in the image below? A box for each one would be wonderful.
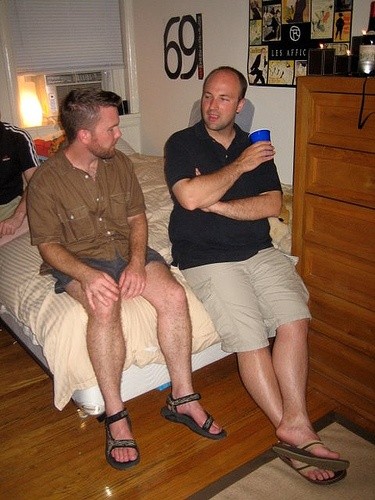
[291,73,375,422]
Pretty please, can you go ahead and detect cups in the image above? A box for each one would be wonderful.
[248,128,271,144]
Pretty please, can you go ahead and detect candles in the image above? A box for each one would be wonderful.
[306,43,335,76]
[351,30,375,77]
[335,49,354,76]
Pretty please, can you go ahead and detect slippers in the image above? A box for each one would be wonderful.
[273,440,350,472]
[277,454,347,485]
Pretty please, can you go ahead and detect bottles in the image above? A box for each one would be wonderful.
[357,0,375,77]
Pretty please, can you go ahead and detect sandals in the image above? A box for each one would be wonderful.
[161,393,227,439]
[96,406,140,470]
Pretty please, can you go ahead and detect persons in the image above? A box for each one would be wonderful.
[335,12,346,40]
[27,85,227,469]
[249,47,267,83]
[161,66,352,485]
[264,8,280,40]
[249,1,262,18]
[0,119,43,252]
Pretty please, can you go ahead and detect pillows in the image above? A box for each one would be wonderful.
[113,138,136,157]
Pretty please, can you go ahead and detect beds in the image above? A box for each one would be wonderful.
[0,151,293,420]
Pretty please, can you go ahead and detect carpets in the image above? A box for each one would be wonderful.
[184,410,375,500]
